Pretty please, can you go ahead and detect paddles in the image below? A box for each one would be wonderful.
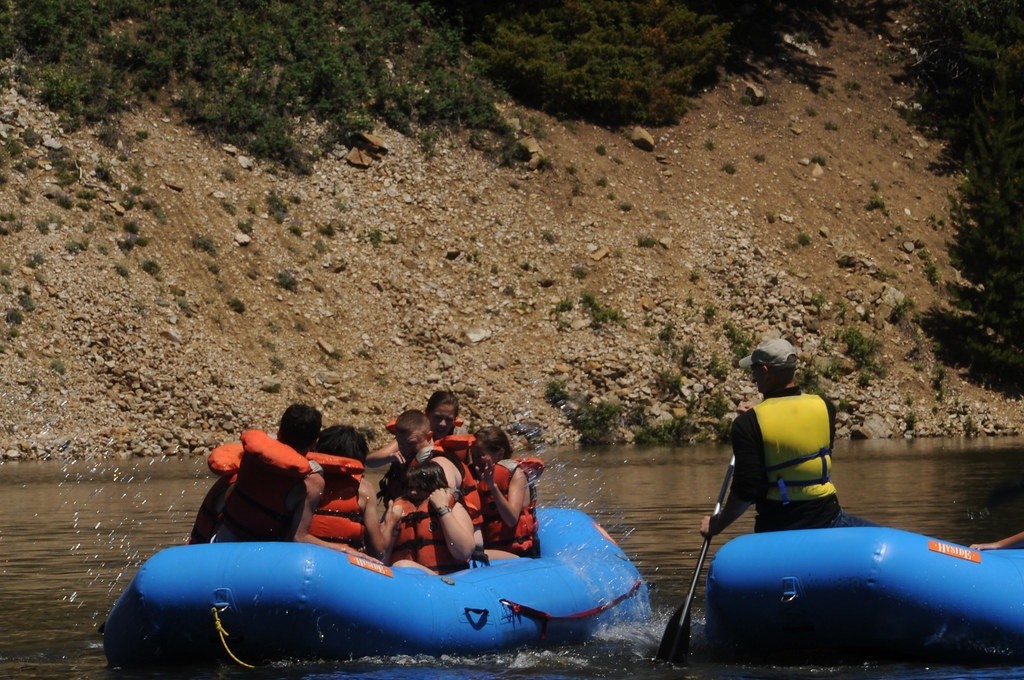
[654,454,737,669]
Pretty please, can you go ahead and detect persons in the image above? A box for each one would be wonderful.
[699,336,883,547]
[314,426,404,569]
[380,407,485,547]
[468,425,545,563]
[187,405,386,564]
[364,390,476,472]
[382,460,482,577]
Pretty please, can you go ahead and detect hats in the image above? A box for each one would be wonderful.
[737,337,800,369]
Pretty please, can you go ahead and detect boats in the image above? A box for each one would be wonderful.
[702,524,1023,667]
[100,504,652,673]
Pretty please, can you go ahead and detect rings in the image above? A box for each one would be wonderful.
[343,547,346,553]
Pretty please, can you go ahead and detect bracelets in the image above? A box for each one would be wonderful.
[435,505,451,517]
